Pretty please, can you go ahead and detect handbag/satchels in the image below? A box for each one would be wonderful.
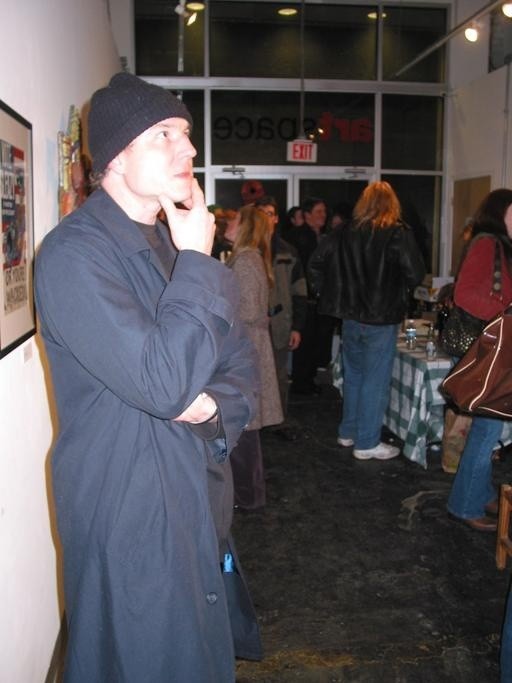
[440,303,512,420]
[439,307,485,355]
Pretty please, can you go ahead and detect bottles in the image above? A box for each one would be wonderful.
[425,321,437,361]
[405,320,417,350]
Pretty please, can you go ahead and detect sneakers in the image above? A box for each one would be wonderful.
[338,438,400,460]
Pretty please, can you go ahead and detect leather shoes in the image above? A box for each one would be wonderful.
[447,501,501,532]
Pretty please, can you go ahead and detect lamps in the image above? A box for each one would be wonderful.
[465,21,480,42]
[175,0,206,27]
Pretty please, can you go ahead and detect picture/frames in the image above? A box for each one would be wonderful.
[0,99,37,360]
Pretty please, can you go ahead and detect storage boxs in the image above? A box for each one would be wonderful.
[414,274,454,302]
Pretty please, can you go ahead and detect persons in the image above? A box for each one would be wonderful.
[256,193,348,436]
[307,180,426,461]
[32,73,265,683]
[445,189,511,531]
[223,203,283,513]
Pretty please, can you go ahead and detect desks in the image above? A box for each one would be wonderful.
[332,318,512,471]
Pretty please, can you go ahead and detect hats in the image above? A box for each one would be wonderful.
[87,72,194,173]
